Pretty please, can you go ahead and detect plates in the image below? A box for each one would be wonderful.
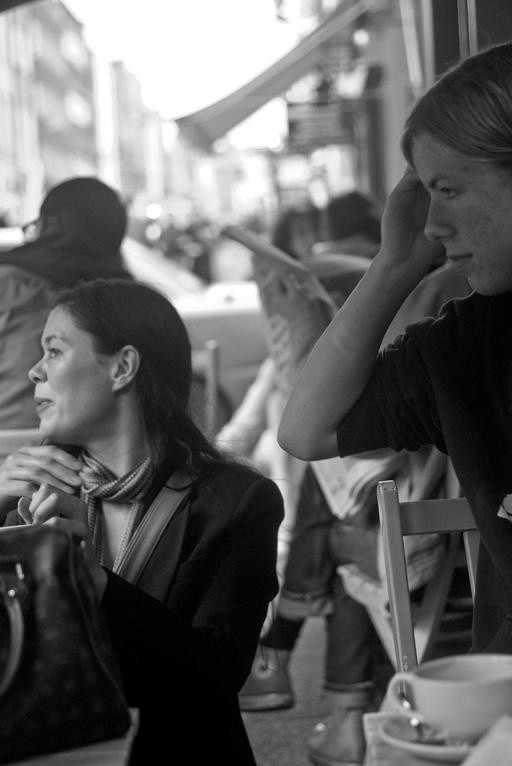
[379,716,474,764]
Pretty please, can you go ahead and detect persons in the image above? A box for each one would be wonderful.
[0,177,136,465]
[237,259,472,766]
[273,204,325,260]
[0,275,285,766]
[308,191,382,277]
[276,42,512,654]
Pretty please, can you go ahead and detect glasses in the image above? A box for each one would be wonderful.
[22,217,42,243]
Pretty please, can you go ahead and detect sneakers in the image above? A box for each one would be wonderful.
[305,707,367,766]
[237,646,295,712]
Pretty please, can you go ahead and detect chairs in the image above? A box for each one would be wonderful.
[0,428,43,462]
[335,454,481,675]
[376,478,479,672]
[191,338,222,445]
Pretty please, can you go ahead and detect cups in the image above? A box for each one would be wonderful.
[385,653,511,741]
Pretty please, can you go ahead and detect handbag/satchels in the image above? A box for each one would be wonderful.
[0,524,132,766]
